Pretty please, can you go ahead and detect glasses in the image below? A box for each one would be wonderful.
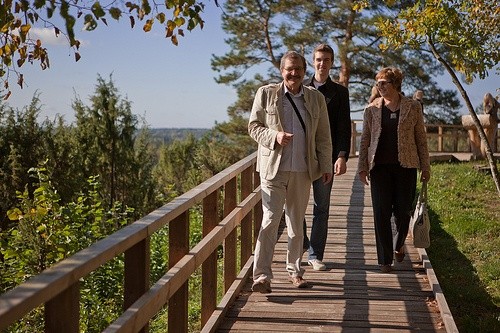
[375,81,392,87]
[282,67,305,72]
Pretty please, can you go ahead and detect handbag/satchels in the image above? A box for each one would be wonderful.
[410,179,431,248]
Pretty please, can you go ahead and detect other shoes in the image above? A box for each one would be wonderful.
[307,258,326,271]
[289,276,308,288]
[380,262,391,273]
[395,250,405,263]
[252,280,272,294]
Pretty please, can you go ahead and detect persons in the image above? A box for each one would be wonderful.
[357,67,430,272]
[484,93,500,153]
[368,83,423,116]
[301,44,352,272]
[248,50,333,294]
